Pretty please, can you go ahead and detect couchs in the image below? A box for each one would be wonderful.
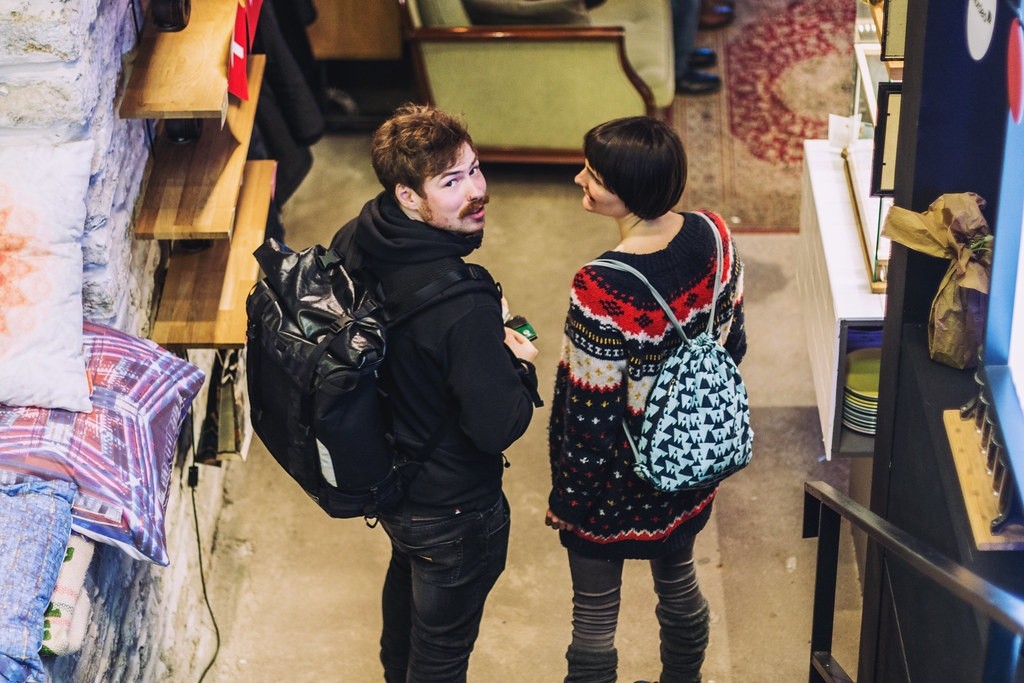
[400,0,677,164]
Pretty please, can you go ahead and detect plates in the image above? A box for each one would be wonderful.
[841,347,882,434]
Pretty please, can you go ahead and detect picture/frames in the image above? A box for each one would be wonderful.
[880,0,908,62]
[870,82,903,197]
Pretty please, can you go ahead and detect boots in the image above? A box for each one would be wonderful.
[564,642,621,682]
[633,600,716,683]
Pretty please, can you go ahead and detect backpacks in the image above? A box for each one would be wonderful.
[242,218,544,530]
[582,210,755,494]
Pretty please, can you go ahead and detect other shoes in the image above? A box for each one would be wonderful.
[697,1,735,30]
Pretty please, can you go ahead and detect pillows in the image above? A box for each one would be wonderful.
[0,469,79,683]
[460,0,593,27]
[0,320,207,566]
[0,139,101,412]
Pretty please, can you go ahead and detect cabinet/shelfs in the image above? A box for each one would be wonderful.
[840,0,910,294]
[119,0,278,349]
[857,0,1024,683]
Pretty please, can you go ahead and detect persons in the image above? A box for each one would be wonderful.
[670,0,735,96]
[545,116,748,683]
[326,106,544,683]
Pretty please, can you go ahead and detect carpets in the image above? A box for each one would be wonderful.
[663,0,857,235]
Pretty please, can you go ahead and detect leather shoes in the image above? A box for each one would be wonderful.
[677,70,719,95]
[688,47,716,69]
